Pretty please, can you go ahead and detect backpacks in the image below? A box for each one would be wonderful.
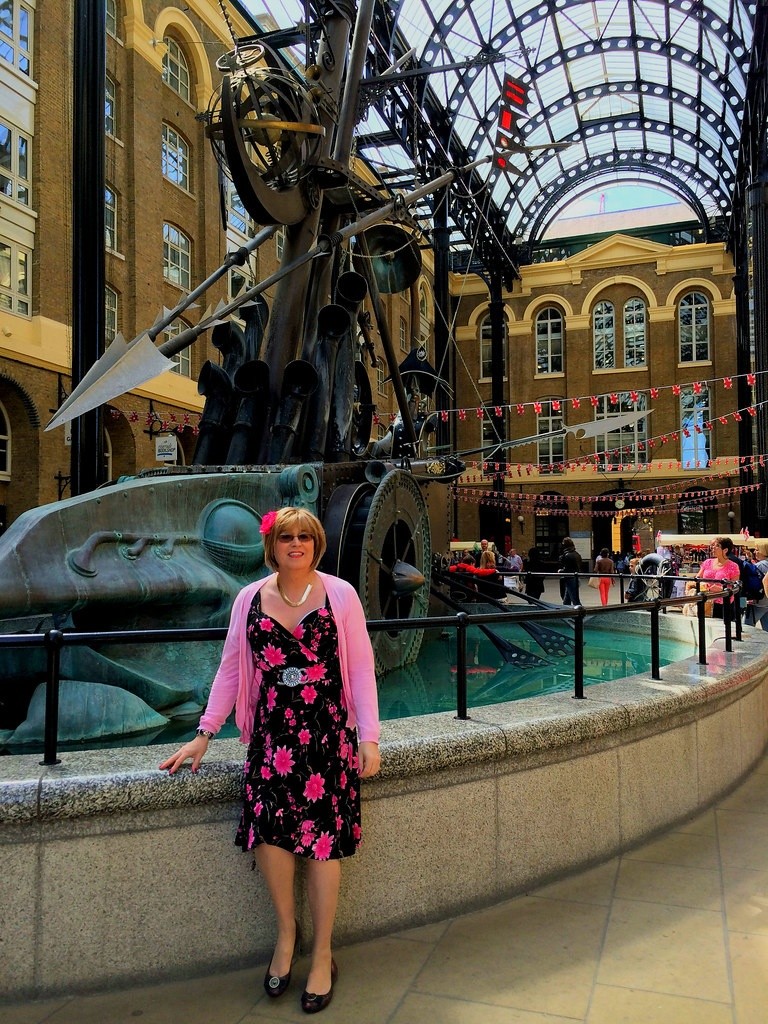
[741,561,765,604]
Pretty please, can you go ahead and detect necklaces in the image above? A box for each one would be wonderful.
[276,574,316,607]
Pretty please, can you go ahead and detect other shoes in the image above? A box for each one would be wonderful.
[519,586,523,592]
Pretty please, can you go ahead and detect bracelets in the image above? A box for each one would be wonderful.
[196,729,214,739]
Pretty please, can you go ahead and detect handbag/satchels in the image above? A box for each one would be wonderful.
[588,576,601,589]
[689,600,714,617]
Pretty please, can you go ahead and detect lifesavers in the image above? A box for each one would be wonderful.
[632,554,673,611]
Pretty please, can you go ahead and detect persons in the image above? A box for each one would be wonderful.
[158,506,381,1014]
[446,539,550,605]
[754,530,761,538]
[560,538,768,630]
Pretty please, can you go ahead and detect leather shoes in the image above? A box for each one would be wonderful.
[264,918,302,997]
[301,957,338,1013]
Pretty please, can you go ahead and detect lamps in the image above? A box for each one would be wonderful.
[517,515,525,534]
[728,511,736,532]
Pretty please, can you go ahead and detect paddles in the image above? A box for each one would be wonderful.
[430,586,557,670]
[435,570,588,657]
[446,571,597,629]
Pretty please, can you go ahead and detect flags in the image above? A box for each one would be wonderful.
[109,413,203,435]
[658,526,751,542]
[373,374,764,516]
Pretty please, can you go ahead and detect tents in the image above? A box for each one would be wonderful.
[656,535,751,564]
[450,541,495,554]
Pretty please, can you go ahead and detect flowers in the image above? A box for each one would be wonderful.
[259,510,277,535]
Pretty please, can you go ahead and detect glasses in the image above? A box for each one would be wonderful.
[628,565,634,567]
[277,534,314,543]
[712,543,722,548]
